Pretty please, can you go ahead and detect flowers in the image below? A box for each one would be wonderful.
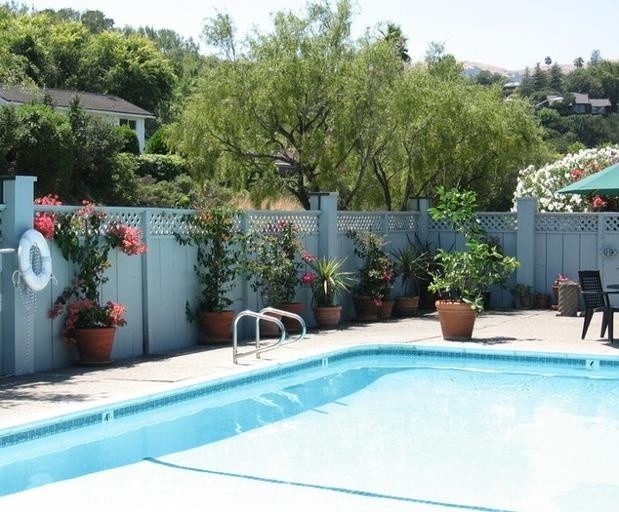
[553,272,569,288]
[34,191,149,345]
[273,219,317,303]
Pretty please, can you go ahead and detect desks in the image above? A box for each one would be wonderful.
[580,285,619,346]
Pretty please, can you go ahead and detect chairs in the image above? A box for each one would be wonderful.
[576,268,619,340]
[603,283,618,344]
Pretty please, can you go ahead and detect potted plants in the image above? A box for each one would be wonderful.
[425,184,520,342]
[512,282,533,310]
[239,227,292,336]
[173,195,246,344]
[341,228,429,324]
[310,252,358,330]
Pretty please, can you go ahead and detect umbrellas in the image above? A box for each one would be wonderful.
[557,162,619,194]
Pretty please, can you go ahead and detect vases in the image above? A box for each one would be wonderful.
[279,303,305,333]
[537,294,551,309]
[73,326,117,365]
[552,284,560,311]
[479,292,490,310]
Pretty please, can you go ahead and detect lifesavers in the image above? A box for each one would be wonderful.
[18,229,52,291]
